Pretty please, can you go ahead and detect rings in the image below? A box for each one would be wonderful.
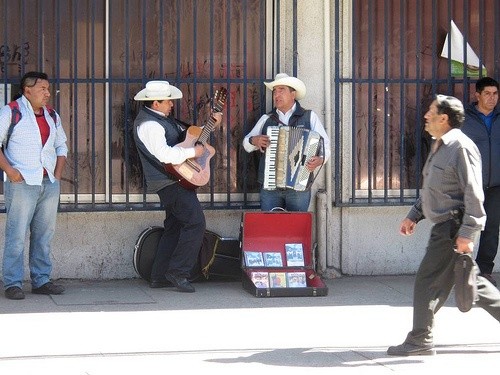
[220,113,223,116]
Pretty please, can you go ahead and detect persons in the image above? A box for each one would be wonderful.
[459,78,500,287]
[242,72,331,212]
[133,80,222,293]
[0,71,69,300]
[387,95,500,357]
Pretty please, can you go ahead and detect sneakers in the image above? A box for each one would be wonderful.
[5,286,24,299]
[164,271,196,293]
[32,282,65,295]
[150,278,175,288]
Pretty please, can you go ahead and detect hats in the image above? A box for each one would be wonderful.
[263,72,307,100]
[133,80,183,101]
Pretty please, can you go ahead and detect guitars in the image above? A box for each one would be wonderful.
[163,86,228,189]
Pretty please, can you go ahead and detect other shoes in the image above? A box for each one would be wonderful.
[387,337,437,356]
